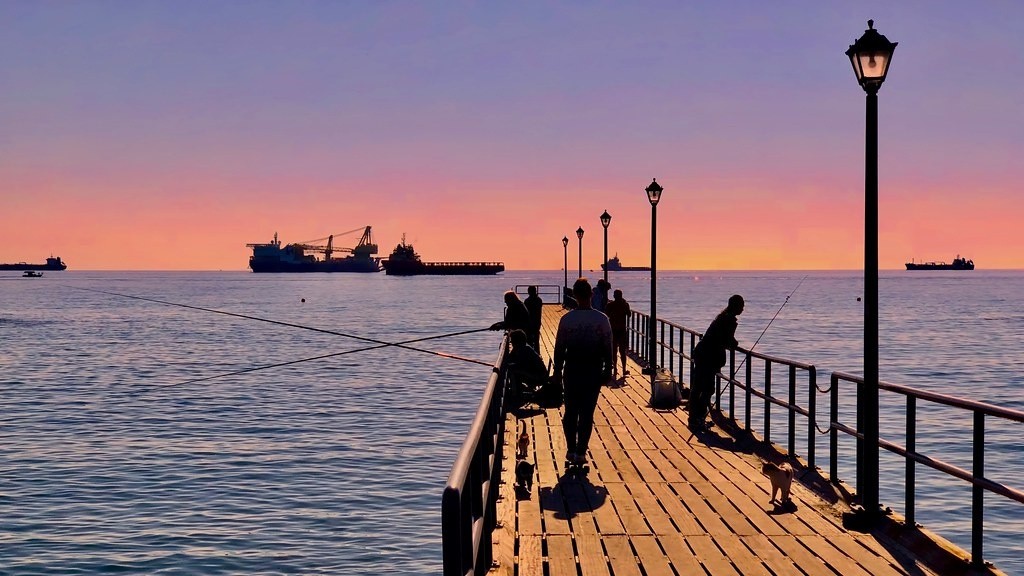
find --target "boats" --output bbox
[380,232,505,276]
[599,250,655,272]
[21,270,43,277]
[244,226,383,273]
[905,252,975,271]
[0,253,71,271]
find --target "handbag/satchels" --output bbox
[533,376,563,408]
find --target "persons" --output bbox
[501,329,548,403]
[553,278,614,463]
[523,286,542,355]
[489,291,530,342]
[687,295,745,431]
[591,280,609,312]
[604,289,632,376]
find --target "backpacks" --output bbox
[649,367,682,410]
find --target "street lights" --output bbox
[645,177,665,382]
[575,226,585,278]
[599,210,613,281]
[562,237,572,292]
[842,21,899,525]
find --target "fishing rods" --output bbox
[685,270,811,446]
[56,279,507,377]
[147,322,503,397]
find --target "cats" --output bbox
[515,459,536,491]
[761,460,794,503]
[518,420,530,458]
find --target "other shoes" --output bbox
[622,370,630,375]
[565,447,589,464]
[687,420,716,437]
